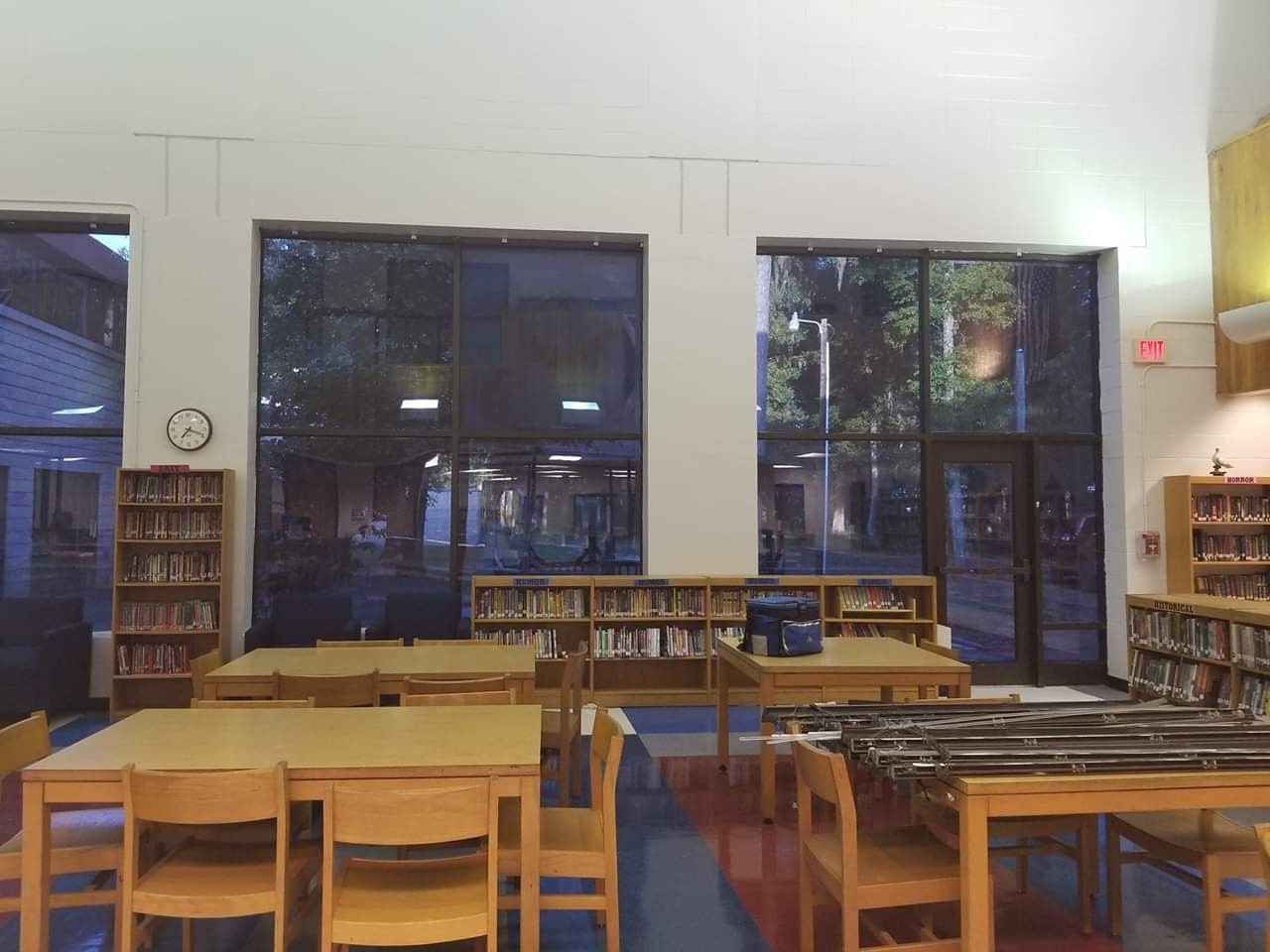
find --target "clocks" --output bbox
[166,408,212,451]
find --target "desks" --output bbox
[924,770,1270,952]
[203,647,536,706]
[717,637,972,823]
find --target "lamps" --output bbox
[1218,301,1270,345]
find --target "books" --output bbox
[1190,494,1270,602]
[116,472,222,674]
[836,586,908,610]
[1126,605,1270,723]
[839,623,885,637]
[751,586,818,599]
[473,587,745,660]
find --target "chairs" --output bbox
[916,638,960,700]
[0,638,624,952]
[0,596,93,715]
[244,591,359,653]
[364,594,471,648]
[786,694,1270,952]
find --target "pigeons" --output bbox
[1211,447,1236,469]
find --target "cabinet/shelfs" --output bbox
[1124,593,1270,719]
[1210,122,1269,396]
[850,483,1063,559]
[110,468,235,724]
[1164,476,1270,594]
[19,704,543,952]
[469,574,938,707]
